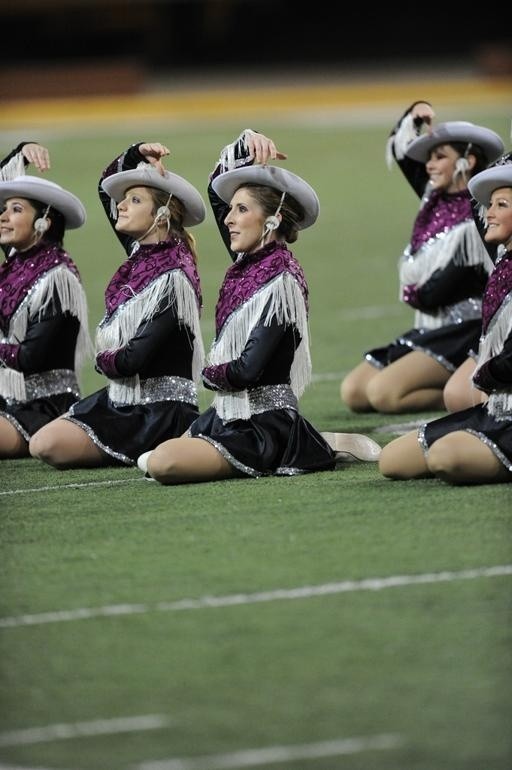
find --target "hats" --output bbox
[101,161,206,228]
[211,162,319,231]
[1,175,86,230]
[467,163,512,209]
[403,121,504,162]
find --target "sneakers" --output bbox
[319,431,382,463]
[137,450,154,475]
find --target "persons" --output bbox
[0,139,93,459]
[28,139,209,477]
[440,348,487,412]
[134,126,381,487]
[339,98,507,416]
[375,150,510,487]
